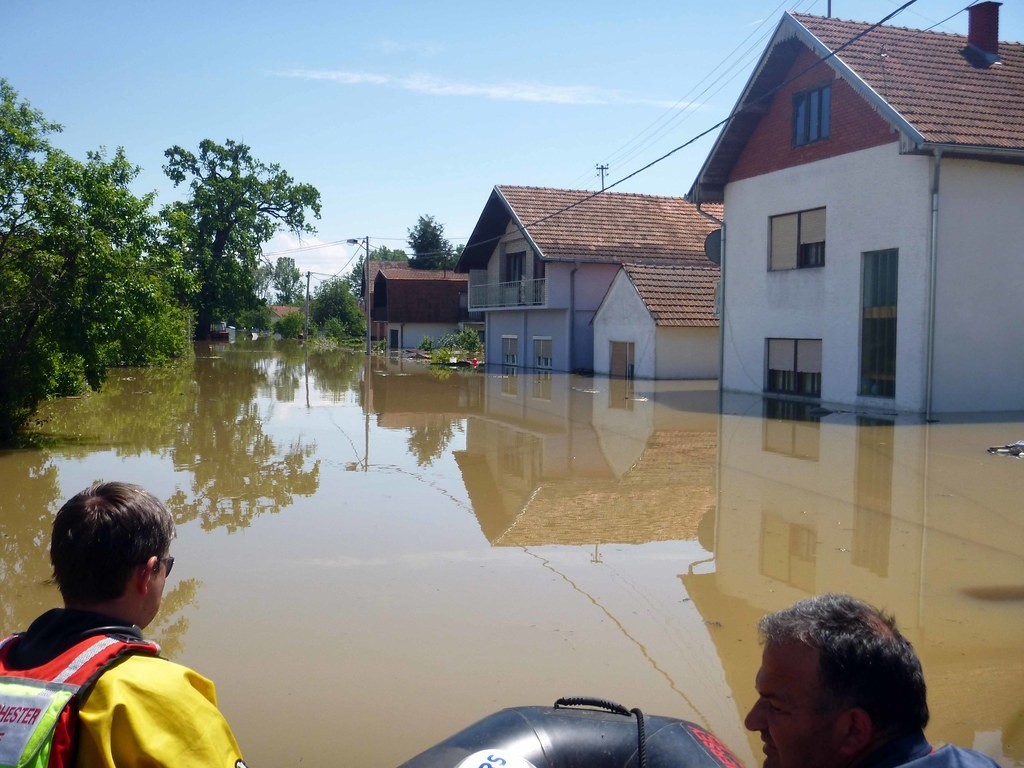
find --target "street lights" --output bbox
[347,236,371,355]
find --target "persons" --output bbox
[0,479,247,768]
[744,591,1002,768]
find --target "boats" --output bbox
[210,321,230,339]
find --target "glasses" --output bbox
[153,555,175,577]
